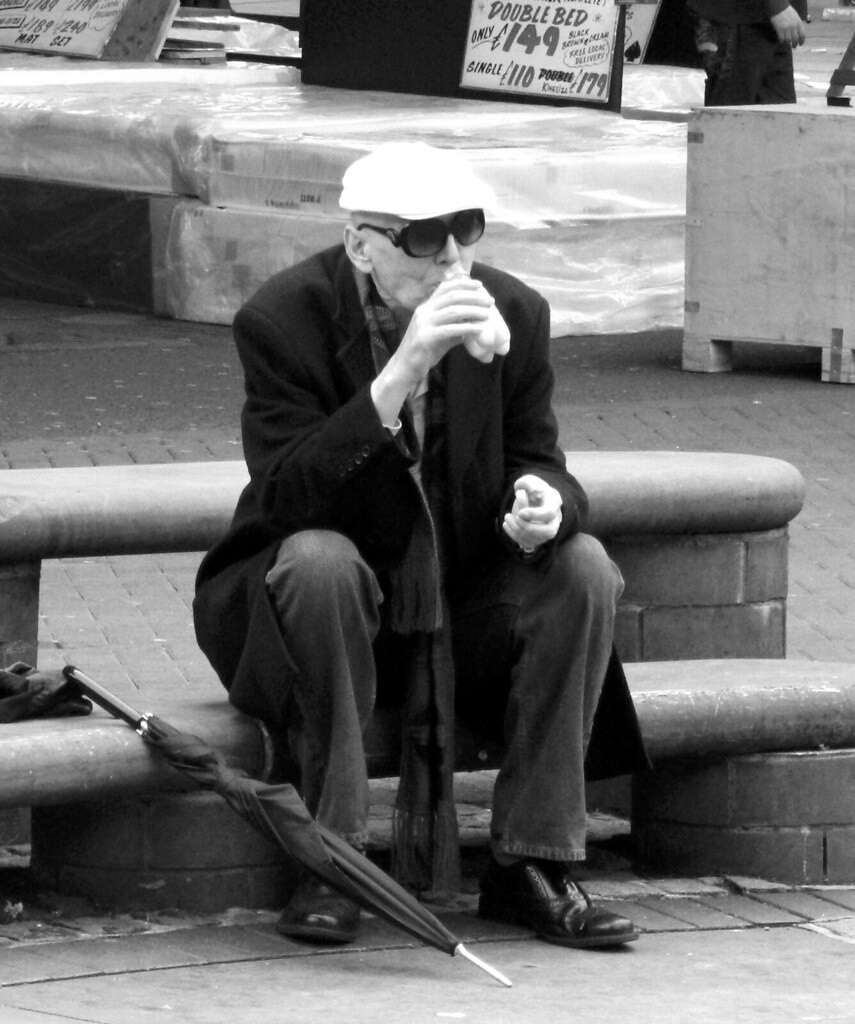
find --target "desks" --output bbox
[684,107,854,384]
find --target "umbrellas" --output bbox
[62,666,517,988]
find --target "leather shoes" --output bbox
[477,857,639,947]
[279,876,362,945]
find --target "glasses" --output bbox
[357,209,485,258]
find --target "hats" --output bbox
[339,142,497,220]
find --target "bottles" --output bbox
[445,264,511,363]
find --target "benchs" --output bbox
[1,451,855,914]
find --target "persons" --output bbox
[193,144,653,949]
[689,0,808,108]
